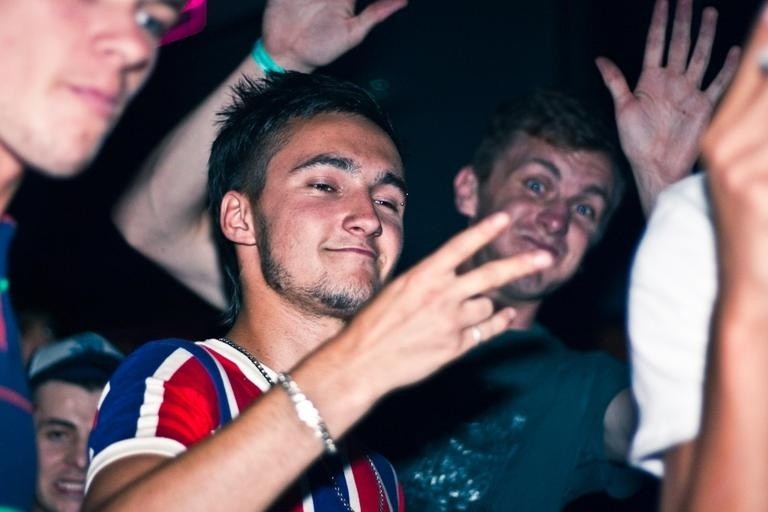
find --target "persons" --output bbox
[2,2,766,511]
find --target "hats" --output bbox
[26,332,125,389]
